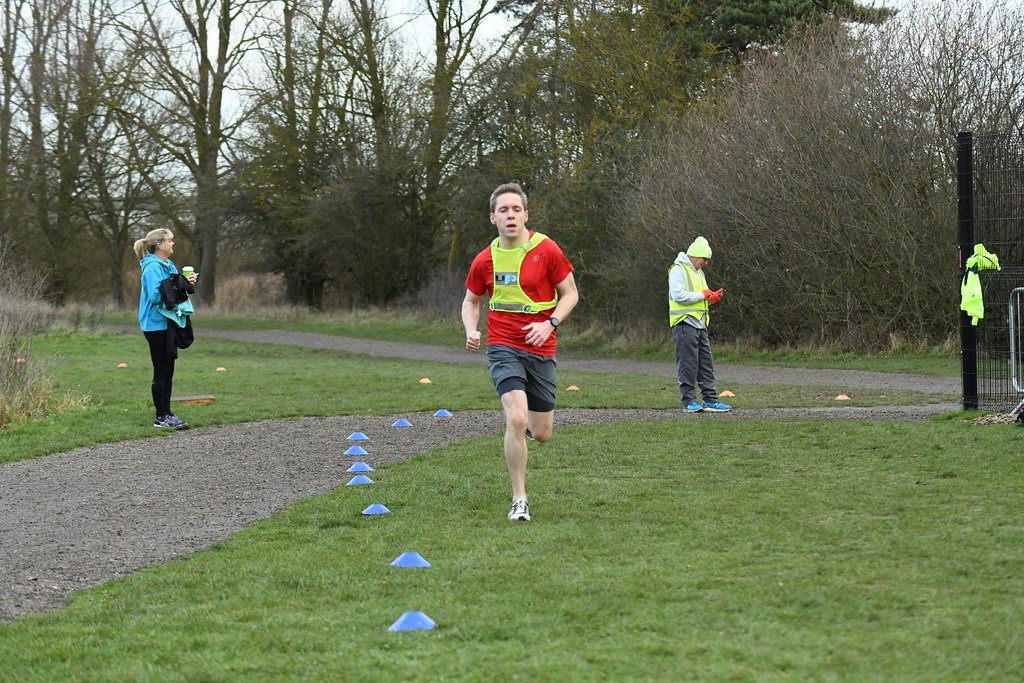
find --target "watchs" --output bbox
[547,317,560,331]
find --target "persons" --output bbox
[134,229,199,429]
[460,184,579,522]
[668,235,733,413]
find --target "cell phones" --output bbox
[717,289,727,297]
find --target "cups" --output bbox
[182,266,195,278]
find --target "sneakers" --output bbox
[702,401,732,412]
[683,401,703,412]
[155,416,188,430]
[524,429,534,440]
[508,497,532,521]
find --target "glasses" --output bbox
[703,257,708,261]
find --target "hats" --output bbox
[687,235,712,258]
